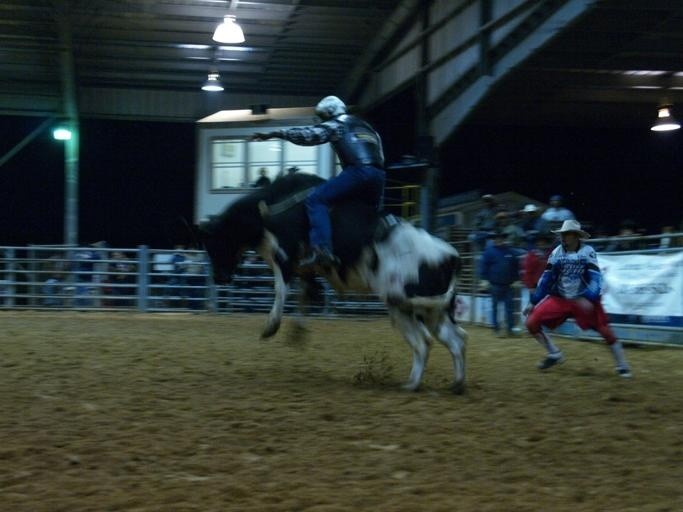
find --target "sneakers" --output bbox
[617,365,631,377]
[536,349,562,369]
[298,248,341,274]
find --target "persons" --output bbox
[473,193,645,340]
[244,95,387,268]
[522,220,632,377]
[256,167,271,185]
[41,242,202,308]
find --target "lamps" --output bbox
[647,90,682,133]
[201,50,224,93]
[212,1,245,45]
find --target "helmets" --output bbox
[314,95,347,118]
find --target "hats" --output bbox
[481,194,591,238]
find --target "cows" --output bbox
[175,173,469,395]
